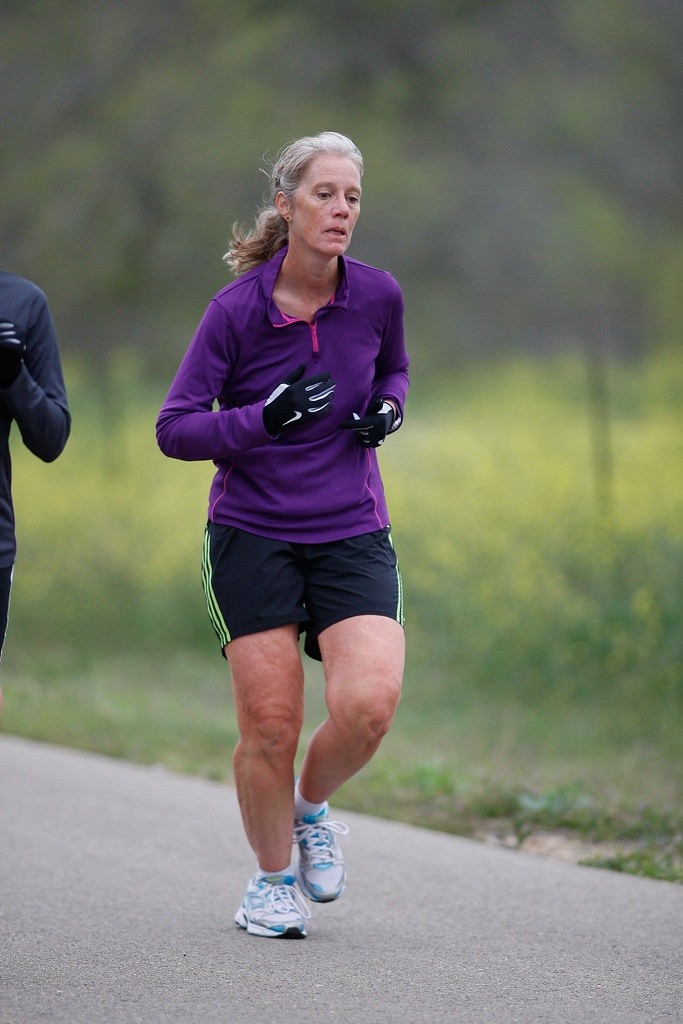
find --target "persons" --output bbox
[1,269,73,656]
[156,130,411,939]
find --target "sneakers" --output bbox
[235,874,312,939]
[293,775,348,903]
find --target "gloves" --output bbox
[0,318,27,389]
[340,398,394,447]
[263,364,337,435]
